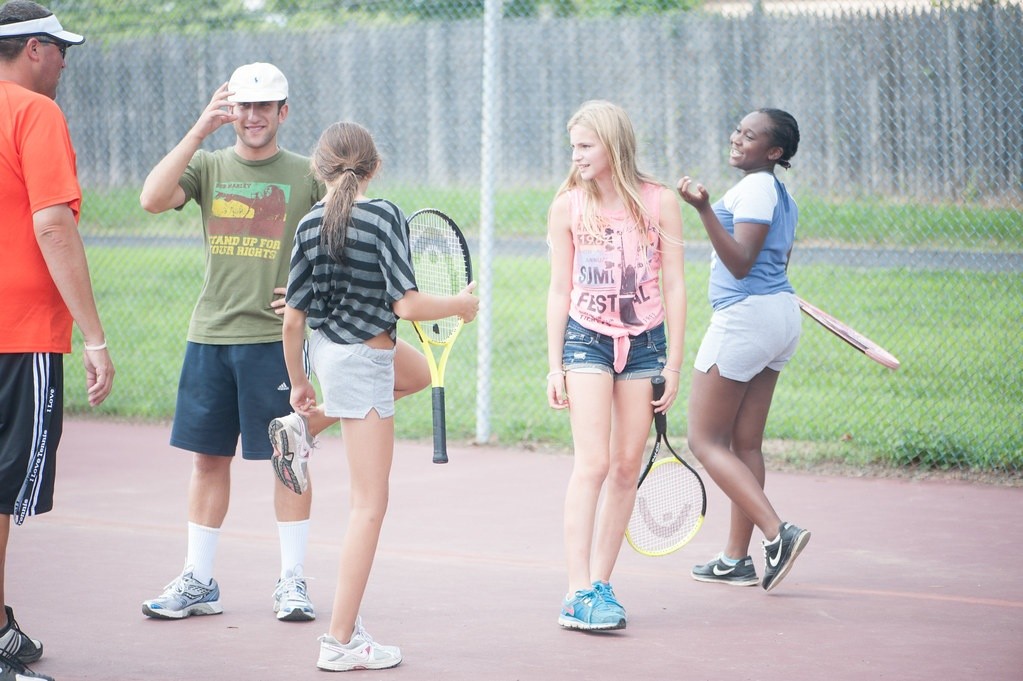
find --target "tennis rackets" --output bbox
[794,294,901,372]
[623,374,710,560]
[405,206,472,466]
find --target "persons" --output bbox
[545,100,687,629]
[268,121,480,671]
[678,109,811,592]
[140,62,326,622]
[0,0,115,681]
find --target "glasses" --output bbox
[22,39,66,60]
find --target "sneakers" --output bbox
[316,615,403,672]
[272,564,316,623]
[761,521,811,591]
[141,557,223,619]
[0,605,43,664]
[691,555,759,587]
[558,587,626,630]
[268,410,323,495]
[593,579,628,617]
[0,648,57,681]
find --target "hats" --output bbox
[227,62,289,102]
[0,13,86,45]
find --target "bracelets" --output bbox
[85,340,107,351]
[547,370,565,379]
